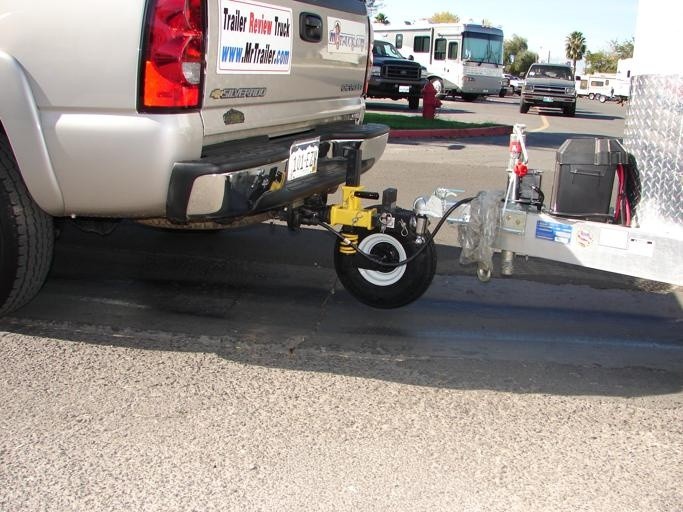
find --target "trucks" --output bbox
[576,59,630,107]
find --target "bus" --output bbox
[361,21,507,102]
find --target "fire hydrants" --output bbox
[418,78,444,120]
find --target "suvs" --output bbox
[366,39,429,110]
[518,62,576,116]
[0,0,391,328]
[502,73,522,96]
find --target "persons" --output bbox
[556,71,564,78]
